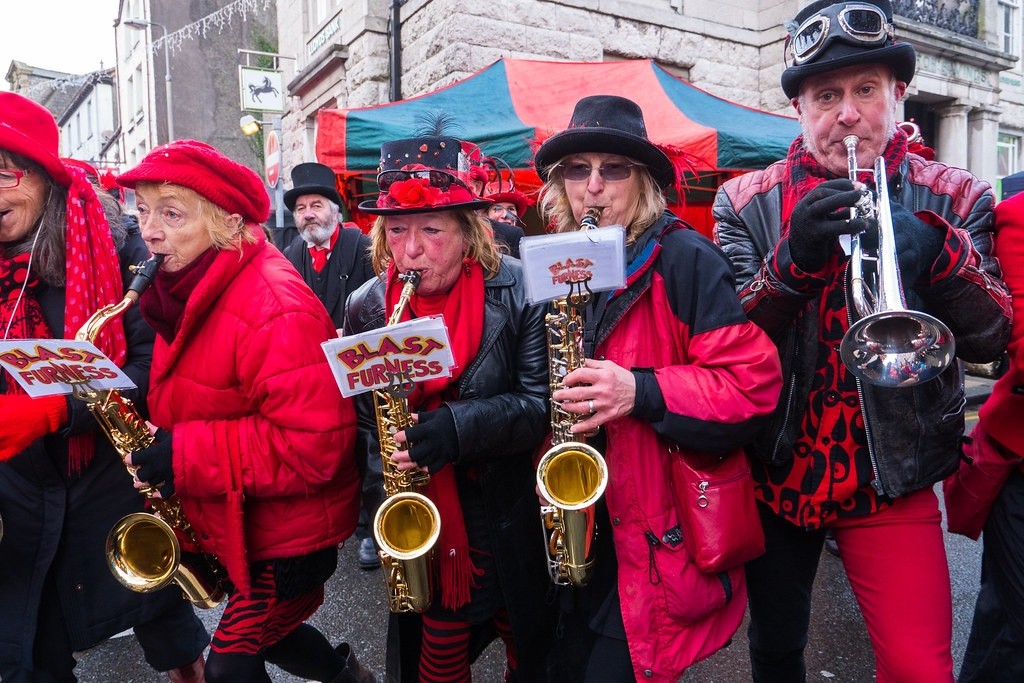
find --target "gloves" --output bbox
[788,178,867,273]
[868,200,943,286]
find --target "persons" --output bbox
[343,105,579,683]
[0,90,210,683]
[279,162,385,567]
[533,97,784,683]
[114,140,378,683]
[712,1,1014,683]
[943,189,1024,683]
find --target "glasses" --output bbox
[559,161,636,181]
[377,169,473,197]
[790,1,894,65]
[0,167,39,187]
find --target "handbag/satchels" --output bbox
[673,448,767,572]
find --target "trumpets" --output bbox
[837,133,956,393]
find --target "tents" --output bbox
[315,57,804,245]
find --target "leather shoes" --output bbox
[358,537,382,567]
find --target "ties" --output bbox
[309,224,340,272]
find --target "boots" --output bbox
[323,641,377,683]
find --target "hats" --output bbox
[781,0,916,101]
[483,181,527,219]
[358,111,491,215]
[284,162,343,213]
[115,139,271,222]
[535,95,674,191]
[0,91,71,185]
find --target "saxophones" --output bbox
[365,269,443,617]
[536,203,614,589]
[67,250,228,613]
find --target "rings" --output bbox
[589,400,594,413]
